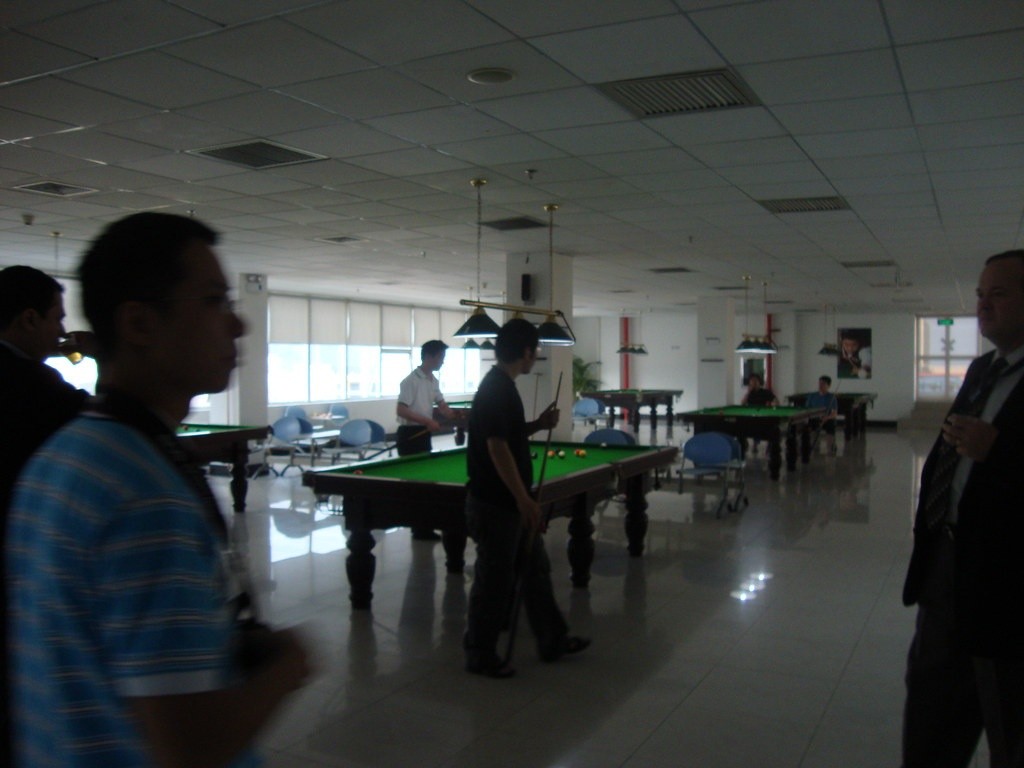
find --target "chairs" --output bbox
[270,398,751,518]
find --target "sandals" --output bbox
[466,656,516,676]
[542,636,590,663]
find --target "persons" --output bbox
[396,340,465,540]
[461,318,594,678]
[841,330,872,376]
[903,249,1024,768]
[0,210,313,768]
[741,373,780,407]
[798,376,838,457]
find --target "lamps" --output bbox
[818,304,842,356]
[734,276,779,354]
[617,308,648,355]
[452,178,577,350]
[247,275,264,294]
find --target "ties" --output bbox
[924,357,1008,532]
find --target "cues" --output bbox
[811,393,836,450]
[502,372,562,665]
[358,410,471,462]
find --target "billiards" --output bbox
[183,425,188,430]
[553,446,561,453]
[574,449,580,456]
[532,452,538,458]
[600,443,607,447]
[579,450,586,457]
[547,450,555,457]
[719,411,724,416]
[559,451,565,457]
[699,412,704,414]
[354,470,363,475]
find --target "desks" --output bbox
[302,440,679,626]
[175,422,269,512]
[432,401,474,446]
[312,414,344,427]
[676,405,828,482]
[786,392,877,441]
[575,388,683,434]
